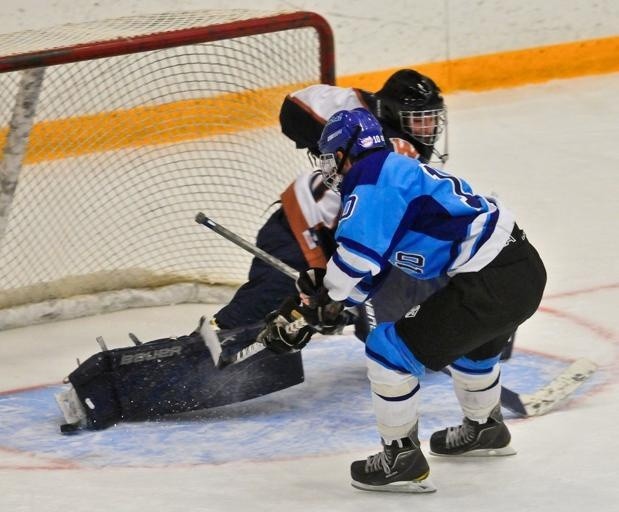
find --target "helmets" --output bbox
[385,69,449,165]
[318,107,386,196]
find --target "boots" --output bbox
[350,420,430,486]
[430,400,511,455]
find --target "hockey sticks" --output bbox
[194,211,596,418]
[199,316,310,369]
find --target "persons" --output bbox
[58,68,450,437]
[255,105,549,485]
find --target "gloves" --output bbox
[262,267,343,357]
